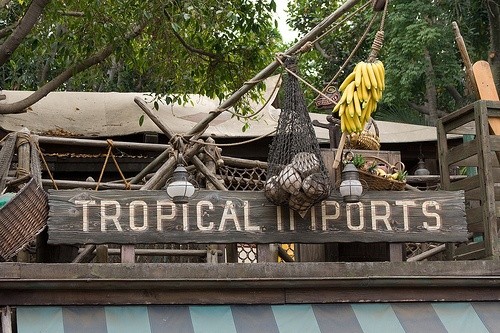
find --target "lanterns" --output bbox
[414,159,430,176]
[160,163,200,204]
[330,161,369,203]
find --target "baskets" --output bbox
[0,175,50,262]
[356,156,407,191]
[345,117,380,150]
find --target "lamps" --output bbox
[339,158,363,203]
[163,152,200,204]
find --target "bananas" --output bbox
[372,168,399,181]
[332,60,385,135]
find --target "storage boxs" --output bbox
[340,150,407,191]
